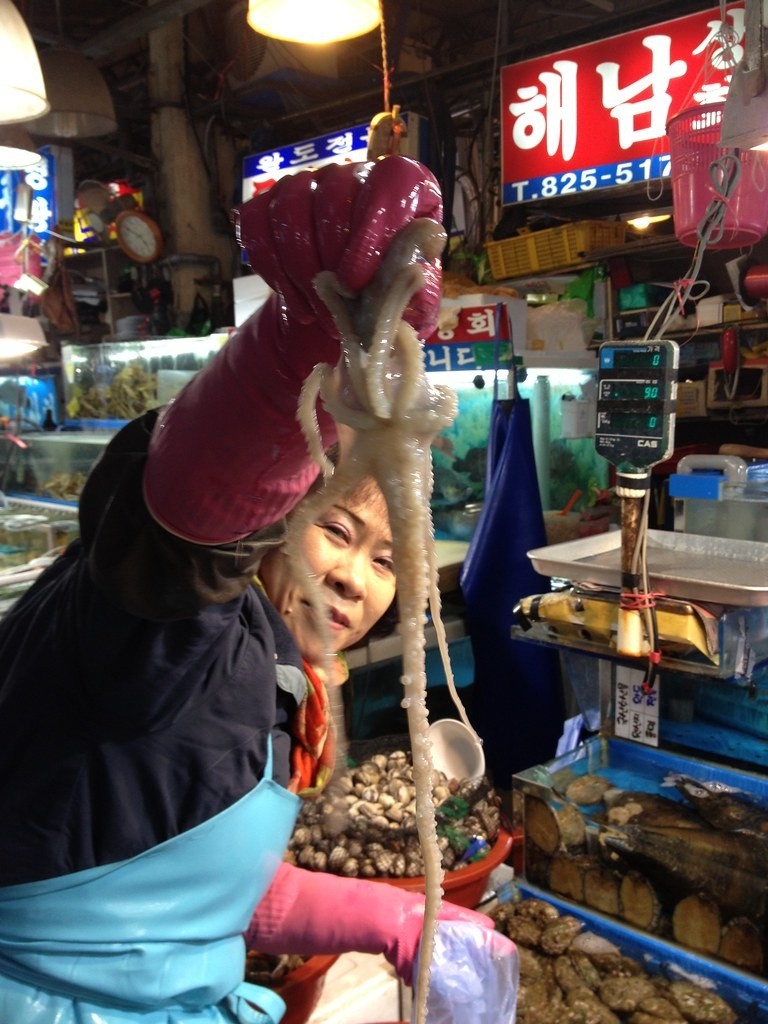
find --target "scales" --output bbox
[526,341,768,685]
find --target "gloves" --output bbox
[148,154,445,545]
[245,863,517,988]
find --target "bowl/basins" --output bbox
[363,829,513,909]
[244,951,340,1024]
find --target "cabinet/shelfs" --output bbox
[603,232,768,437]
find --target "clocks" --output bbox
[116,210,163,263]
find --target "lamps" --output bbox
[0,314,49,359]
[0,0,118,167]
[246,0,383,45]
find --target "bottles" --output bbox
[562,393,577,435]
[150,289,167,336]
[213,285,223,328]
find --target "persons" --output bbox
[0,153,516,1024]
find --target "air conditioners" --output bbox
[223,3,337,94]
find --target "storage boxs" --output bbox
[301,366,768,1024]
[706,365,768,410]
[482,221,627,283]
[0,333,238,620]
[619,283,670,308]
[620,306,663,338]
[667,292,733,332]
[676,379,708,417]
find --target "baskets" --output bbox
[482,220,629,279]
